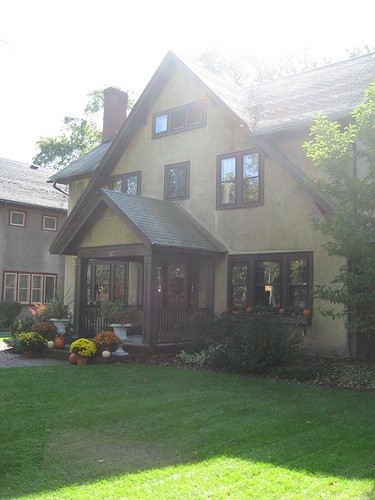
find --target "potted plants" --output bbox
[99,296,143,342]
[246,303,256,314]
[42,298,73,336]
[47,333,55,349]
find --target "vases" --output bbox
[23,348,33,359]
[54,335,68,349]
[69,354,78,364]
[303,306,312,316]
[101,350,111,358]
[279,308,287,315]
[76,355,90,366]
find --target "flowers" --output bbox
[70,337,98,357]
[31,320,58,338]
[17,331,48,351]
[92,330,122,353]
